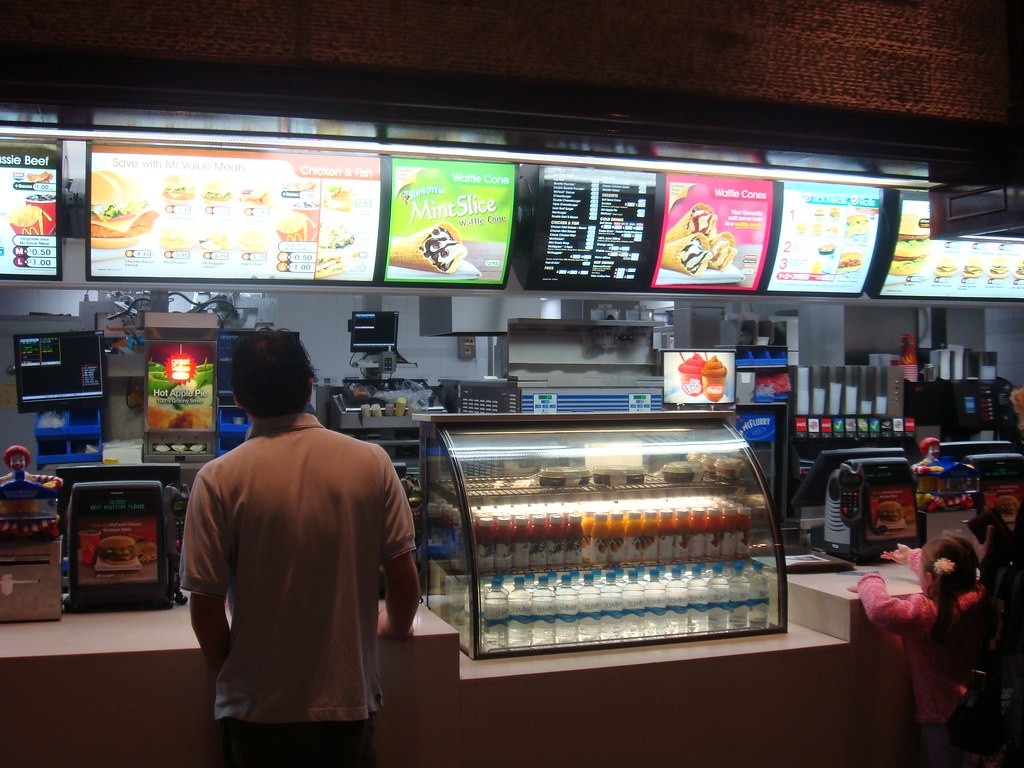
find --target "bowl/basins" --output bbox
[756,337,770,345]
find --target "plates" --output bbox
[877,518,907,530]
[94,557,143,572]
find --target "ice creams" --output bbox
[389,222,467,274]
[708,231,738,271]
[665,202,718,243]
[661,233,710,276]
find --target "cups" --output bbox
[685,451,744,483]
[371,404,382,416]
[590,307,604,320]
[898,332,919,382]
[604,308,619,320]
[79,530,101,565]
[924,362,935,382]
[385,404,394,416]
[293,207,320,241]
[625,309,640,321]
[639,309,655,321]
[679,373,726,403]
[361,404,371,416]
[25,199,56,234]
[395,397,407,416]
[870,497,879,525]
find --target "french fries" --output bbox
[277,213,305,233]
[10,204,40,226]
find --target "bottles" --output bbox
[449,569,605,650]
[670,335,674,349]
[600,560,771,643]
[436,502,584,572]
[580,494,752,567]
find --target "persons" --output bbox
[910,438,944,511]
[961,388,1024,768]
[847,535,991,768]
[179,328,420,768]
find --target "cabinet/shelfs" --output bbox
[410,411,789,661]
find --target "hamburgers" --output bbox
[838,253,862,273]
[797,207,840,235]
[889,214,930,276]
[198,225,232,252]
[162,222,196,249]
[161,175,194,204]
[239,176,273,208]
[879,501,902,525]
[936,258,958,277]
[91,171,159,248]
[202,180,232,204]
[97,535,137,564]
[239,228,273,252]
[989,257,1010,278]
[847,216,869,239]
[1014,258,1024,279]
[962,259,983,277]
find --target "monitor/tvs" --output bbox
[938,441,1011,465]
[792,448,904,507]
[350,311,398,353]
[56,463,181,537]
[13,330,106,414]
